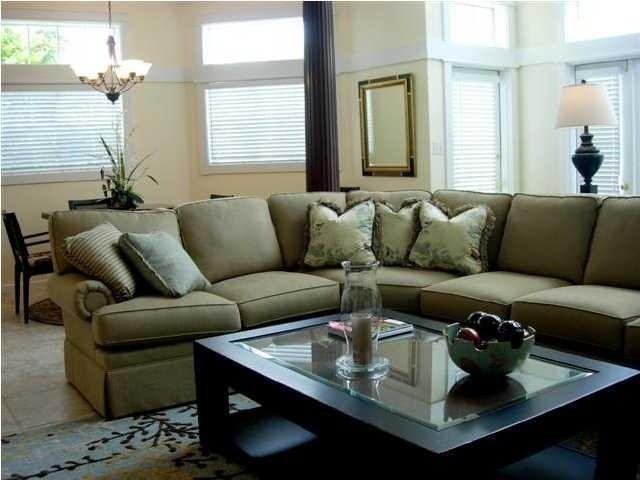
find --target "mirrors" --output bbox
[357,73,418,178]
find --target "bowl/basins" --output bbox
[444,373,526,420]
[442,321,536,375]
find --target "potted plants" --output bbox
[99,114,159,210]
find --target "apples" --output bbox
[456,312,529,349]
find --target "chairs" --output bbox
[1,209,54,323]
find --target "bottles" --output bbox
[344,372,385,404]
[337,260,390,374]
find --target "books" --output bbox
[328,316,414,342]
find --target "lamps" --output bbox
[68,1,152,105]
[555,79,619,194]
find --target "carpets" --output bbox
[30,297,64,326]
[1,390,262,480]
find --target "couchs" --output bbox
[45,189,639,421]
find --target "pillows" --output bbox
[295,195,374,271]
[419,195,494,274]
[121,232,214,298]
[57,224,136,295]
[374,198,417,267]
